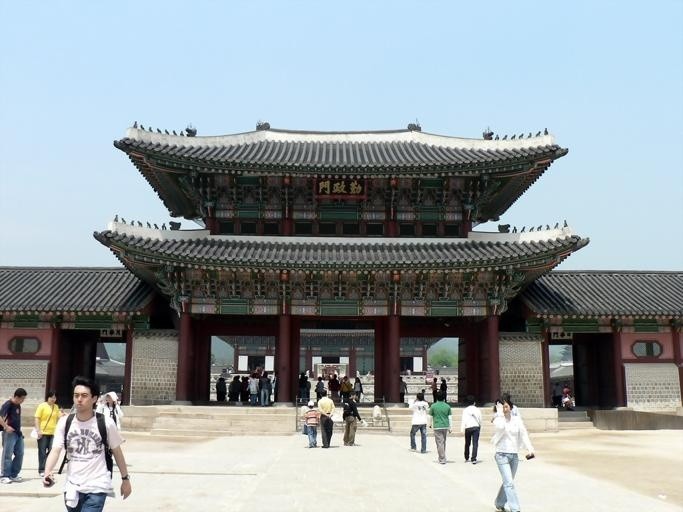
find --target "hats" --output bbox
[307,401,315,407]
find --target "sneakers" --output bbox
[472,459,478,464]
[39,471,45,477]
[407,447,449,465]
[495,503,507,512]
[10,476,23,482]
[0,476,12,484]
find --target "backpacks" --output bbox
[64,412,115,481]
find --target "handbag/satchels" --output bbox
[0,423,5,432]
[302,423,309,435]
[30,428,42,440]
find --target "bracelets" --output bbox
[3,425,7,430]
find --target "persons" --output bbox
[41,376,131,511]
[489,395,535,512]
[427,389,452,464]
[299,372,368,448]
[215,372,271,406]
[552,389,563,406]
[429,376,439,400]
[420,388,430,404]
[439,381,449,402]
[562,384,575,397]
[32,390,66,476]
[0,387,27,483]
[95,391,123,479]
[459,397,481,463]
[400,376,408,401]
[562,394,573,407]
[490,393,521,423]
[408,392,430,453]
[491,399,503,415]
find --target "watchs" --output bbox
[121,474,130,480]
